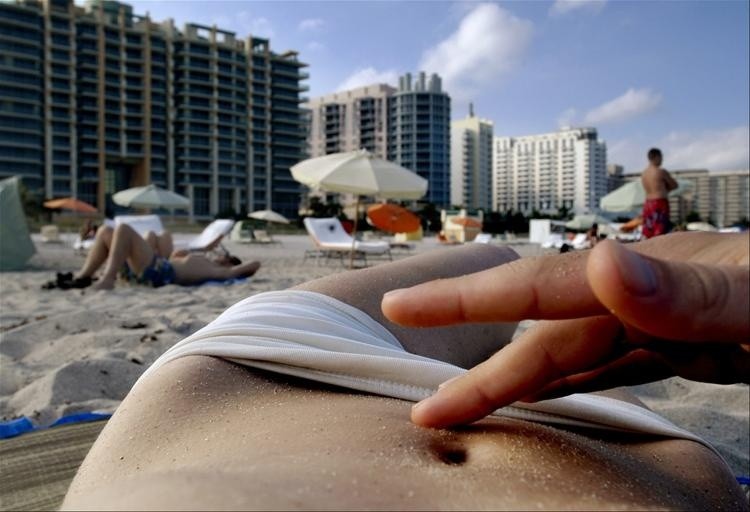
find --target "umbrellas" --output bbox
[291,147,428,270]
[450,215,484,243]
[112,183,190,215]
[43,195,98,213]
[248,209,289,226]
[366,201,422,252]
[600,176,687,214]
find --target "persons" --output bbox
[57,229,750,512]
[586,223,597,245]
[72,222,262,293]
[639,146,679,240]
[144,231,174,257]
[555,227,578,247]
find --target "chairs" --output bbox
[303,215,393,268]
[253,230,284,248]
[474,231,493,244]
[188,218,235,250]
[541,232,592,251]
[114,213,165,240]
